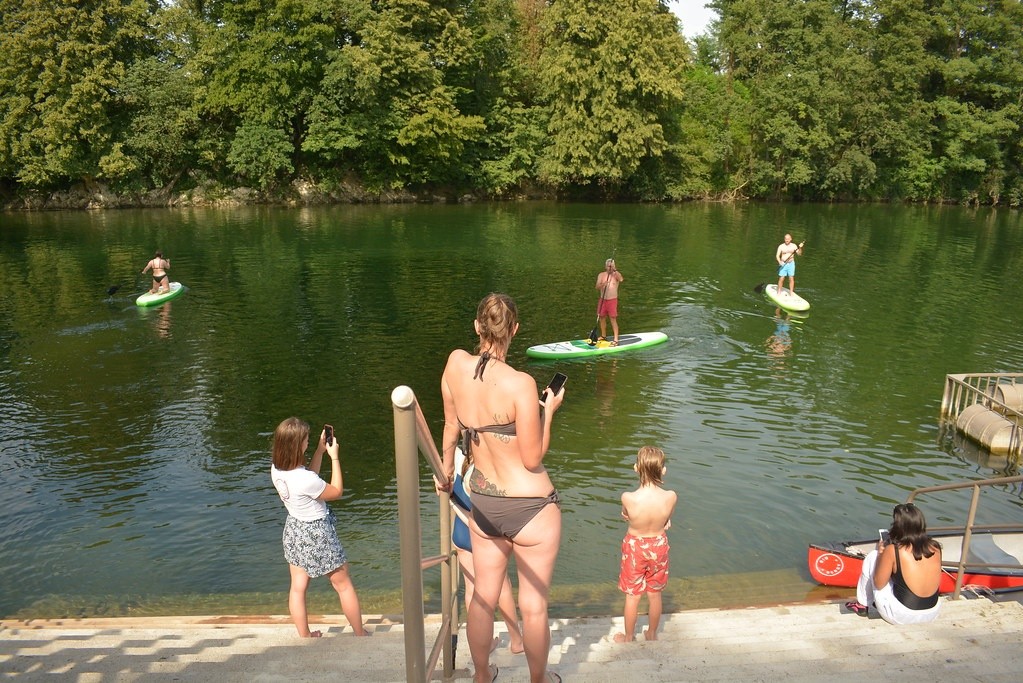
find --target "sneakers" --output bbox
[872,602,876,609]
[845,602,869,617]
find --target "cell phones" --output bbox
[324,424,334,446]
[879,529,889,541]
[539,372,568,407]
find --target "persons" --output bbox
[142,251,171,295]
[433,294,565,683]
[845,503,942,625]
[613,446,677,643]
[270,417,369,637]
[595,259,624,347]
[776,234,803,296]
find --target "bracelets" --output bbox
[614,270,618,272]
[331,459,339,460]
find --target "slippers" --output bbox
[473,666,498,683]
[546,672,563,683]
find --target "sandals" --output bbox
[609,341,619,347]
[597,336,606,340]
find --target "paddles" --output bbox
[587,247,617,347]
[105,274,142,295]
[754,238,808,294]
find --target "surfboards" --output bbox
[765,282,811,313]
[135,281,184,307]
[525,330,669,360]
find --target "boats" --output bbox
[808,522,1023,599]
